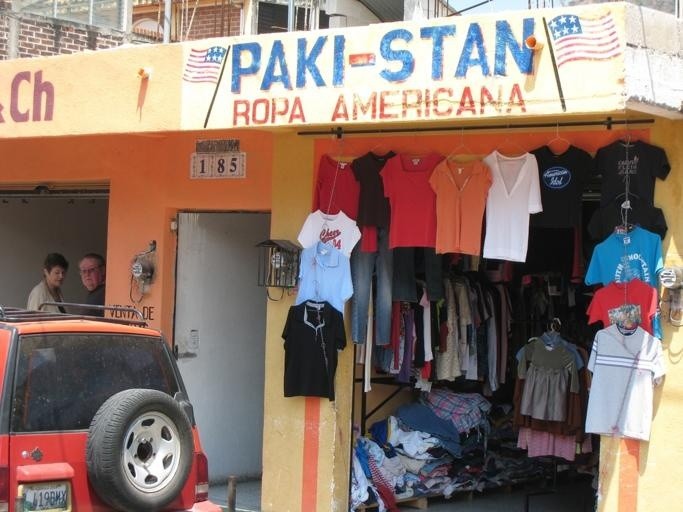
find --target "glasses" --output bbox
[79,264,104,274]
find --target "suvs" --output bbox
[0,294,224,511]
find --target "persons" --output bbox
[77,252,104,316]
[24,252,71,313]
